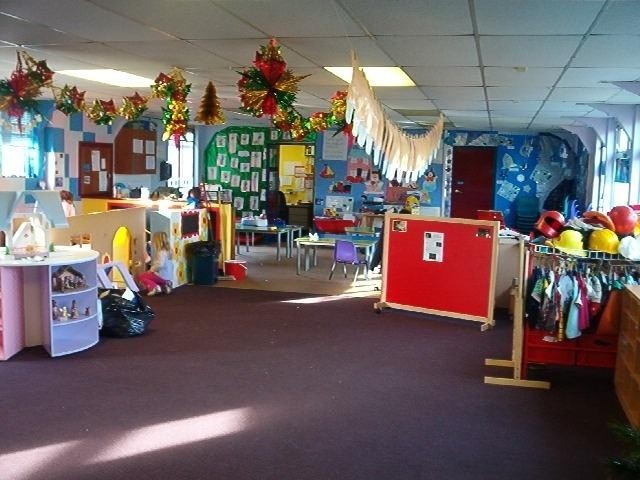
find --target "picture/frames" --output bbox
[235,222,303,262]
[294,236,377,276]
[306,233,381,240]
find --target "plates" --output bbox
[612,284,640,434]
[0,247,99,362]
[521,325,617,380]
[289,205,311,229]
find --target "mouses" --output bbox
[537,255,636,279]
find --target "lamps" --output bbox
[474,209,505,231]
[515,192,541,234]
[329,240,368,282]
[265,190,289,224]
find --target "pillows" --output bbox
[535,204,640,262]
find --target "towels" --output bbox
[186,240,222,286]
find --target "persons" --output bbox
[60,191,75,217]
[137,232,174,296]
